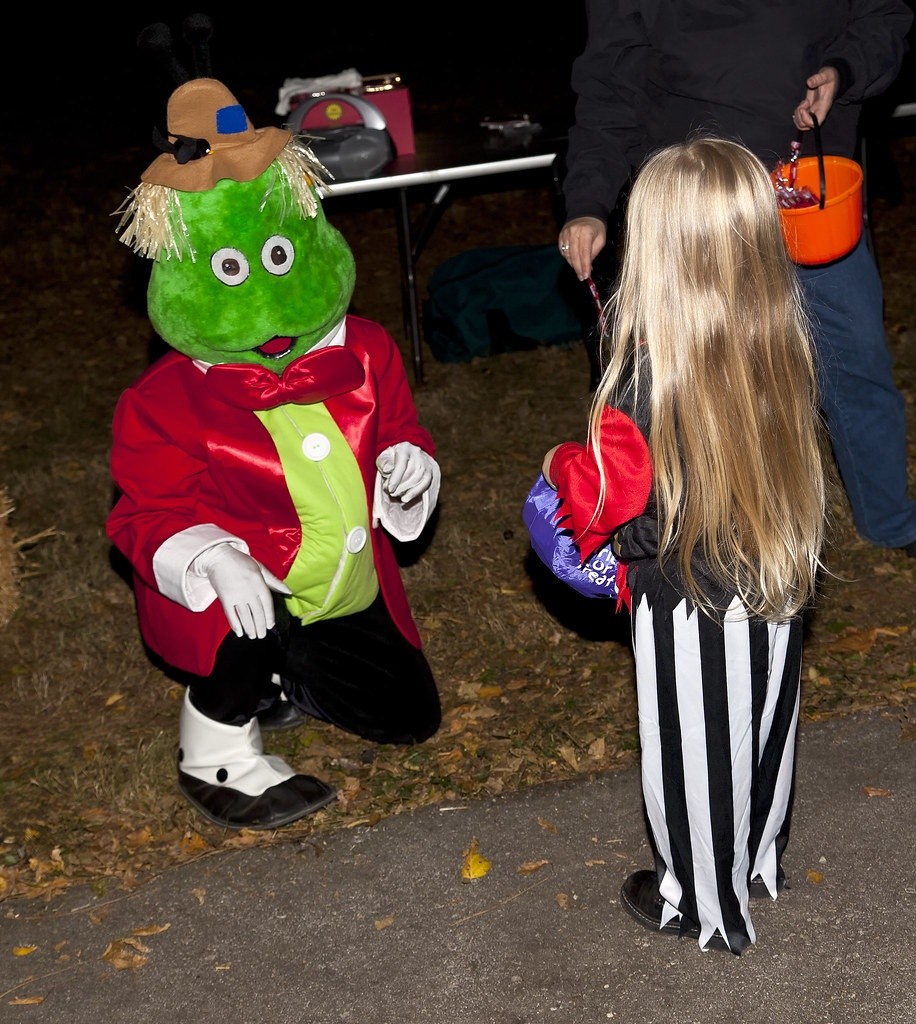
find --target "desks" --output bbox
[313,153,558,383]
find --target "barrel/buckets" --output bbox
[767,154,864,266]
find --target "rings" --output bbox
[562,244,569,250]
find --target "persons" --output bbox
[559,0,916,562]
[541,138,826,958]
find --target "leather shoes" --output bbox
[748,863,786,899]
[619,869,731,951]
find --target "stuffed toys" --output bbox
[104,78,442,831]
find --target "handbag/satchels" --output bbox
[521,470,619,599]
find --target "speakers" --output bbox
[286,94,397,182]
